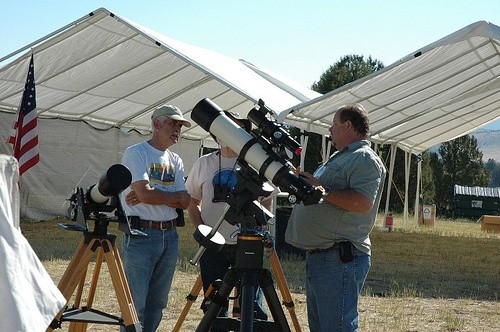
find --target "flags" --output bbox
[6,54,40,175]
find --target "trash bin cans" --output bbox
[418,204,423,224]
[423,204,436,227]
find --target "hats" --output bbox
[210,109,252,143]
[152,105,191,128]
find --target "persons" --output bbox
[119,105,192,332]
[0,152,67,332]
[284,104,386,332]
[184,111,274,332]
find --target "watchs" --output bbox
[324,186,332,195]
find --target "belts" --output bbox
[139,221,177,231]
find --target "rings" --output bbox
[133,198,137,202]
